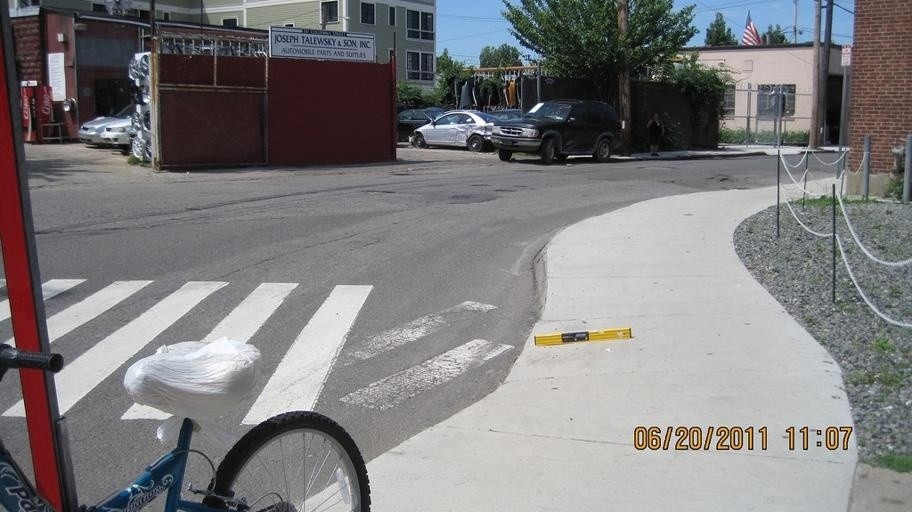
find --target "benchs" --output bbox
[741,12,762,47]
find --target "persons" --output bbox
[646,112,680,157]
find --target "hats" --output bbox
[77,100,137,156]
[397,98,627,164]
